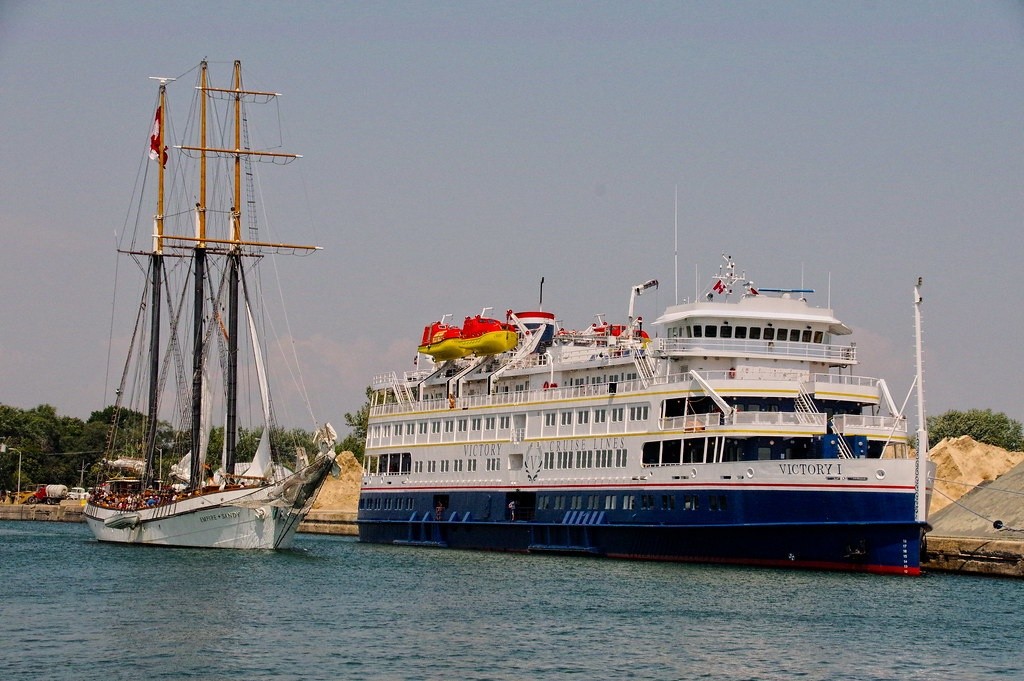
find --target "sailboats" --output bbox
[82,57,343,551]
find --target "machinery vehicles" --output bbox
[5,483,68,506]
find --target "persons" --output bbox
[89,464,246,511]
[33,485,46,501]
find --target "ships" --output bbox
[356,182,933,576]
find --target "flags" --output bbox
[148,105,168,169]
[713,280,726,295]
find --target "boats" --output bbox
[416,314,473,362]
[459,306,518,357]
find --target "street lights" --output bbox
[9,447,21,505]
